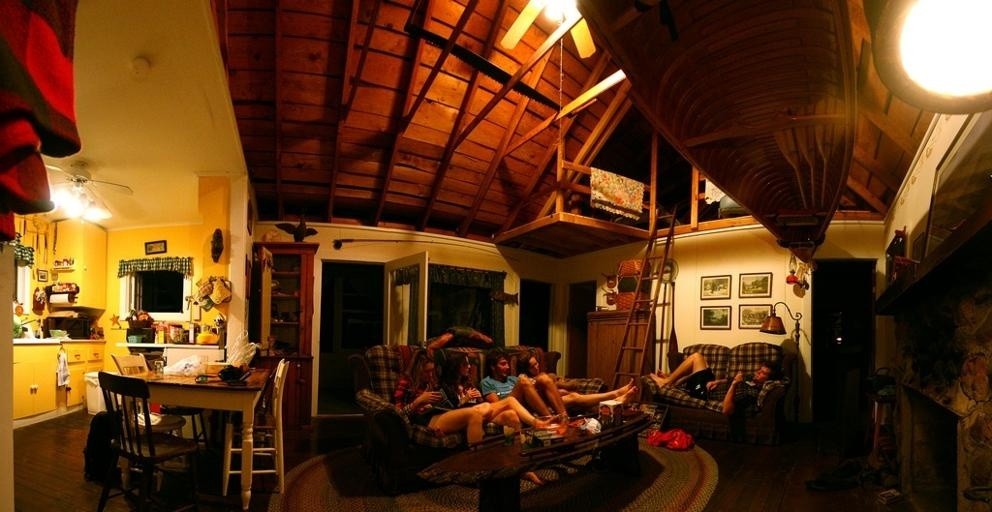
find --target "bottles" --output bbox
[34,325,44,339]
[153,320,220,345]
[54,258,68,268]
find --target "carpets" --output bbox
[269,431,719,512]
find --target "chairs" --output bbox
[223,357,291,497]
[138,350,209,449]
[111,351,186,437]
[96,370,197,512]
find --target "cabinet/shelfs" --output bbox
[57,339,107,421]
[10,333,61,433]
[247,240,321,458]
[585,310,657,402]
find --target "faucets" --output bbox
[19,316,37,326]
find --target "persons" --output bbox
[649,351,782,417]
[393,347,492,444]
[439,351,561,488]
[479,348,569,421]
[514,351,640,411]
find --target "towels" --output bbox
[57,353,71,386]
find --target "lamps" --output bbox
[760,297,803,445]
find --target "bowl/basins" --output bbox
[206,361,232,374]
[127,319,153,328]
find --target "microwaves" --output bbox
[46,316,92,338]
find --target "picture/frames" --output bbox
[739,303,775,330]
[144,239,166,255]
[36,269,48,282]
[700,275,732,300]
[738,272,773,297]
[698,305,732,332]
[919,113,992,263]
[913,232,925,261]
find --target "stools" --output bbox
[863,391,898,458]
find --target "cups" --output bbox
[155,360,164,379]
[504,426,515,447]
[520,428,533,448]
[199,354,209,374]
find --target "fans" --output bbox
[42,156,135,226]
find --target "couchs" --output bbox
[348,343,608,494]
[638,334,795,449]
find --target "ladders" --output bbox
[609,204,678,393]
